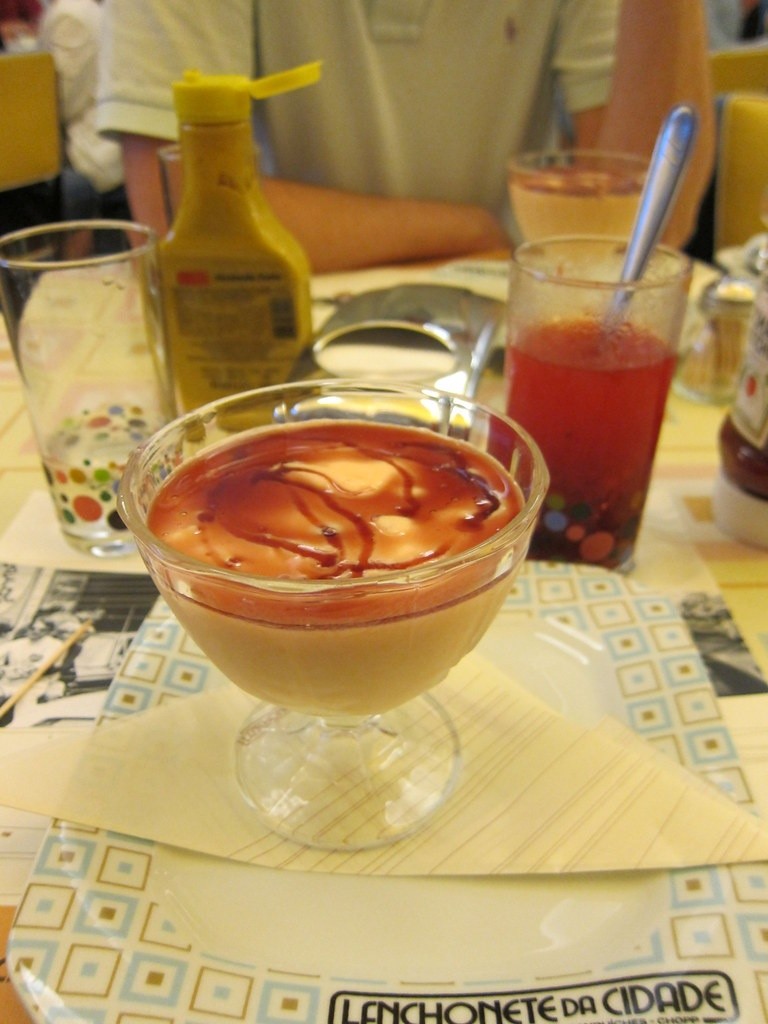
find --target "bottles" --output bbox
[138,58,323,426]
[712,265,768,553]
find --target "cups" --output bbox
[0,216,173,559]
[510,234,695,574]
[151,141,196,228]
[671,260,758,403]
[503,150,649,253]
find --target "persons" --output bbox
[94,0,716,276]
[0,1,121,261]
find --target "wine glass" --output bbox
[117,376,550,851]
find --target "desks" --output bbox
[0,245,768,1024]
